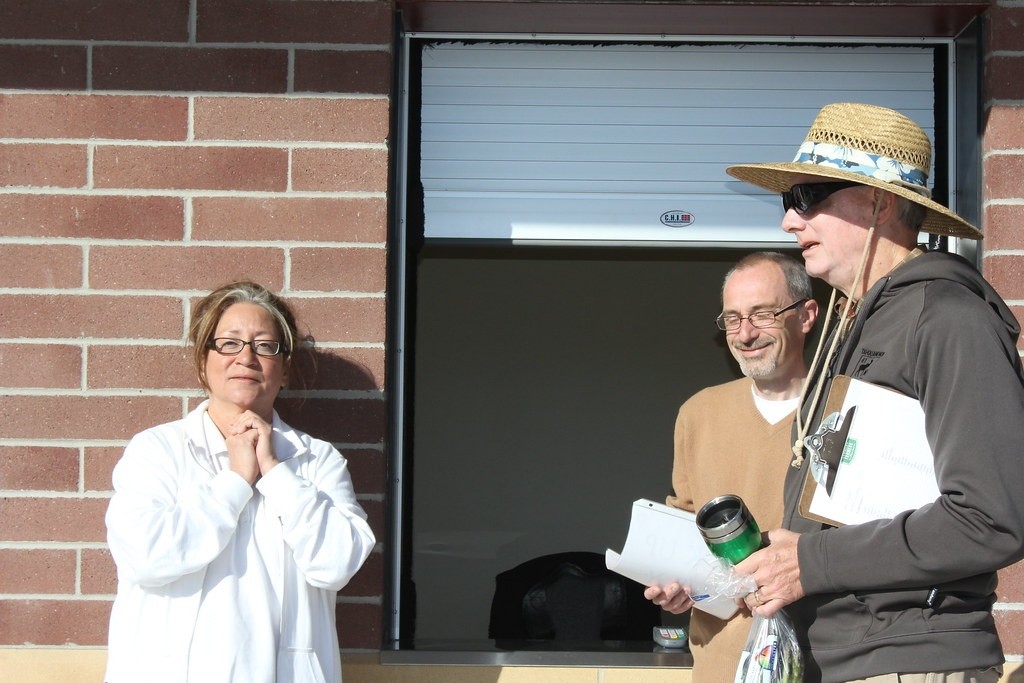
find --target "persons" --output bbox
[105,278,376,683]
[725,104,1024,683]
[644,251,818,683]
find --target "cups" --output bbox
[696,494,767,567]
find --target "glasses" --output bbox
[780,181,865,217]
[205,337,287,356]
[714,297,809,331]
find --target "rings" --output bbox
[755,591,765,605]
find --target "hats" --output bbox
[726,103,986,241]
